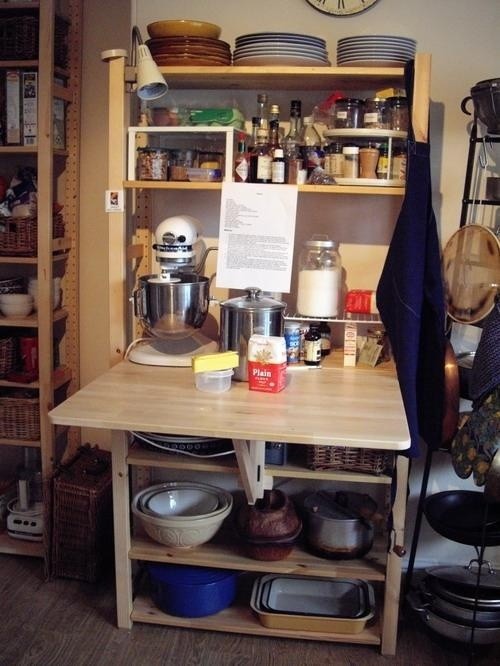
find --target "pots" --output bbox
[424,489,500,544]
[221,287,285,381]
[461,77,499,133]
[303,492,373,557]
[409,562,500,640]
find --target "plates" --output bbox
[234,34,331,65]
[338,36,416,65]
[129,431,237,458]
[149,21,230,66]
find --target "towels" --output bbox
[462,303,499,410]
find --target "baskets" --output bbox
[0,337,18,380]
[306,444,396,476]
[0,8,68,62]
[0,398,64,440]
[1,215,66,255]
[48,446,113,583]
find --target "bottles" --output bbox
[234,130,249,182]
[268,103,282,158]
[322,141,330,175]
[256,90,269,139]
[329,141,343,178]
[295,232,341,317]
[390,143,407,178]
[282,98,305,183]
[341,142,360,178]
[333,97,365,129]
[360,95,385,129]
[377,142,390,180]
[303,323,321,365]
[283,322,301,364]
[136,144,173,181]
[384,93,410,131]
[248,113,262,182]
[270,147,286,183]
[250,139,272,183]
[168,147,199,180]
[299,114,323,178]
[320,319,331,356]
[195,146,224,180]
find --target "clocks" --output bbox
[305,0,381,20]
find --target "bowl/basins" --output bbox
[2,293,30,319]
[131,481,234,549]
[1,277,26,293]
[26,276,60,312]
[250,574,376,633]
[194,369,232,391]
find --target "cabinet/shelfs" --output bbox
[0,0,84,585]
[109,51,434,658]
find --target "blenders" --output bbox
[127,213,217,369]
